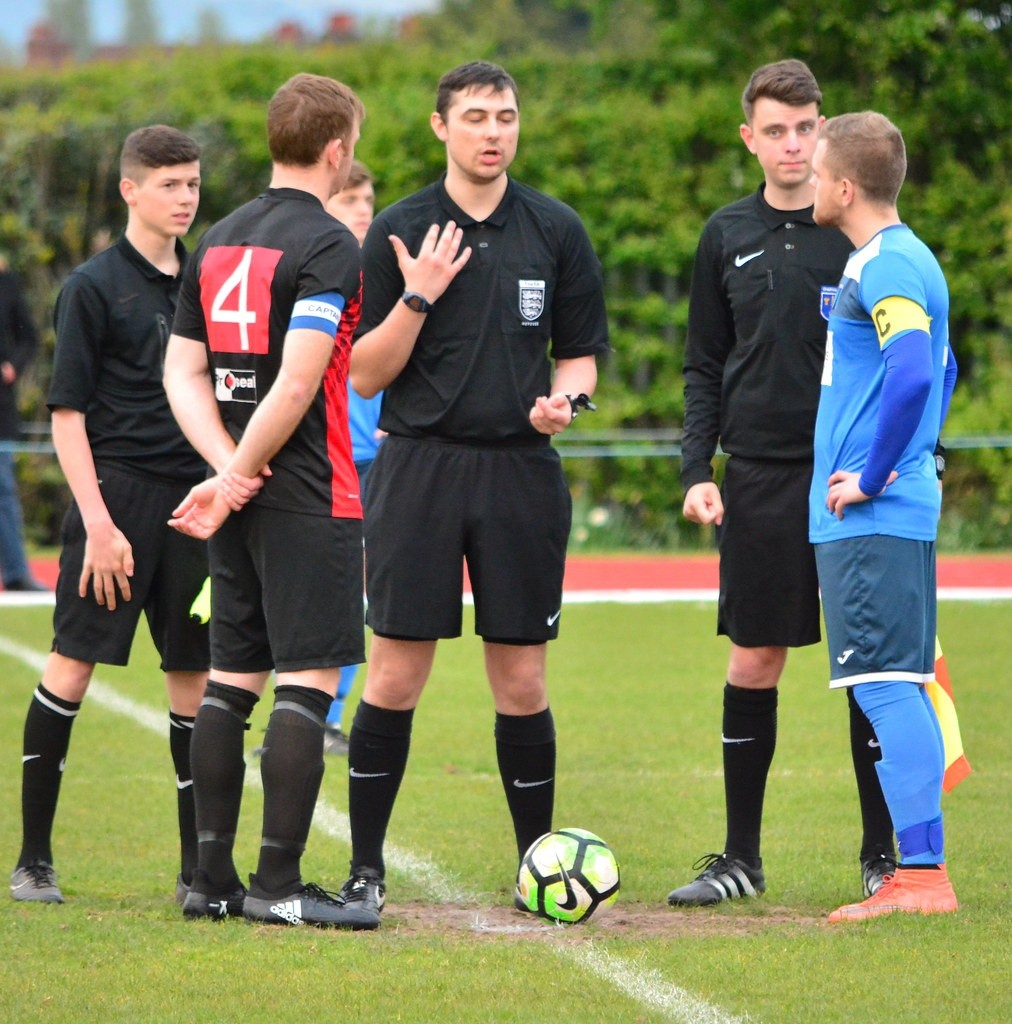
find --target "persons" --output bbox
[161,72,381,932]
[0,265,47,591]
[339,59,612,915]
[810,111,959,922]
[10,122,208,904]
[321,167,389,757]
[665,58,946,905]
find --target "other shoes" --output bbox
[4,576,49,593]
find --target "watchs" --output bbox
[401,290,434,313]
[934,456,945,471]
[566,393,597,426]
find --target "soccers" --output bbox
[515,827,622,928]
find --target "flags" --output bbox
[924,635,976,793]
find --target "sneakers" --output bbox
[828,867,959,924]
[181,869,251,920]
[241,872,381,928]
[323,723,350,755]
[9,859,65,903]
[334,861,388,930]
[859,849,899,898]
[174,871,193,906]
[512,862,563,923]
[665,851,766,907]
